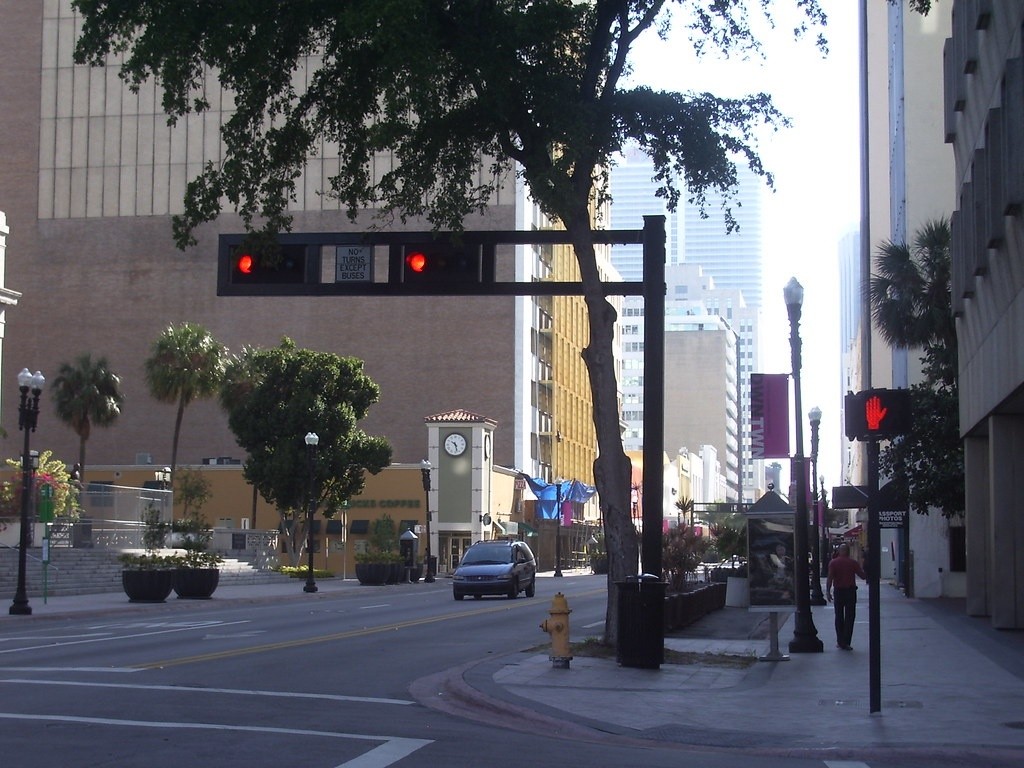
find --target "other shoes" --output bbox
[842,645,853,650]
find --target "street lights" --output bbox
[807,403,828,607]
[420,458,436,584]
[554,475,563,577]
[302,430,320,593]
[783,275,824,653]
[9,368,46,615]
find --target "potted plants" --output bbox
[118,554,179,603]
[355,552,394,587]
[410,559,425,584]
[172,550,224,600]
[388,554,407,586]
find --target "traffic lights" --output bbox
[400,243,478,287]
[859,386,894,436]
[229,247,304,285]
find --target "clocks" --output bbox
[446,433,465,456]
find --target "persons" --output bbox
[69,463,81,490]
[826,541,868,650]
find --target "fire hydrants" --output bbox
[539,592,573,669]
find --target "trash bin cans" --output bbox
[424,555,438,576]
[612,573,670,670]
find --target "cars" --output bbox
[452,538,536,601]
